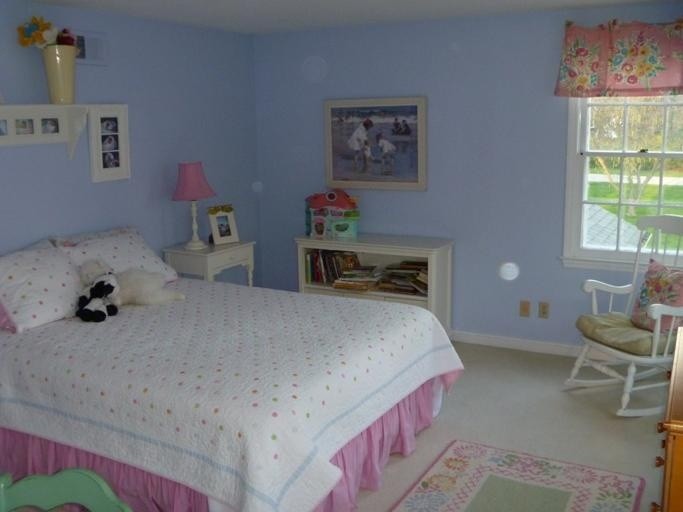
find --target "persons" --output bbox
[217,222,231,237]
[0,117,118,168]
[336,108,411,174]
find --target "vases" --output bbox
[37,42,85,109]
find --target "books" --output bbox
[305,249,428,296]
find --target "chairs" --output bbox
[552,213,683,420]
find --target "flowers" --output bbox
[13,12,98,53]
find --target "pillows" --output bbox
[68,219,181,309]
[0,233,93,336]
[630,258,683,332]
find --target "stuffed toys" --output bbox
[76,273,119,323]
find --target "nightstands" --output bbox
[158,238,262,292]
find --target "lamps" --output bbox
[165,150,223,258]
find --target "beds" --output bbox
[0,220,478,512]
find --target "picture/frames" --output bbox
[318,92,430,196]
[0,100,70,147]
[84,101,134,184]
[205,202,241,246]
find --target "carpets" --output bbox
[377,435,648,511]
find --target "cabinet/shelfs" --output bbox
[289,230,452,343]
[648,327,681,510]
